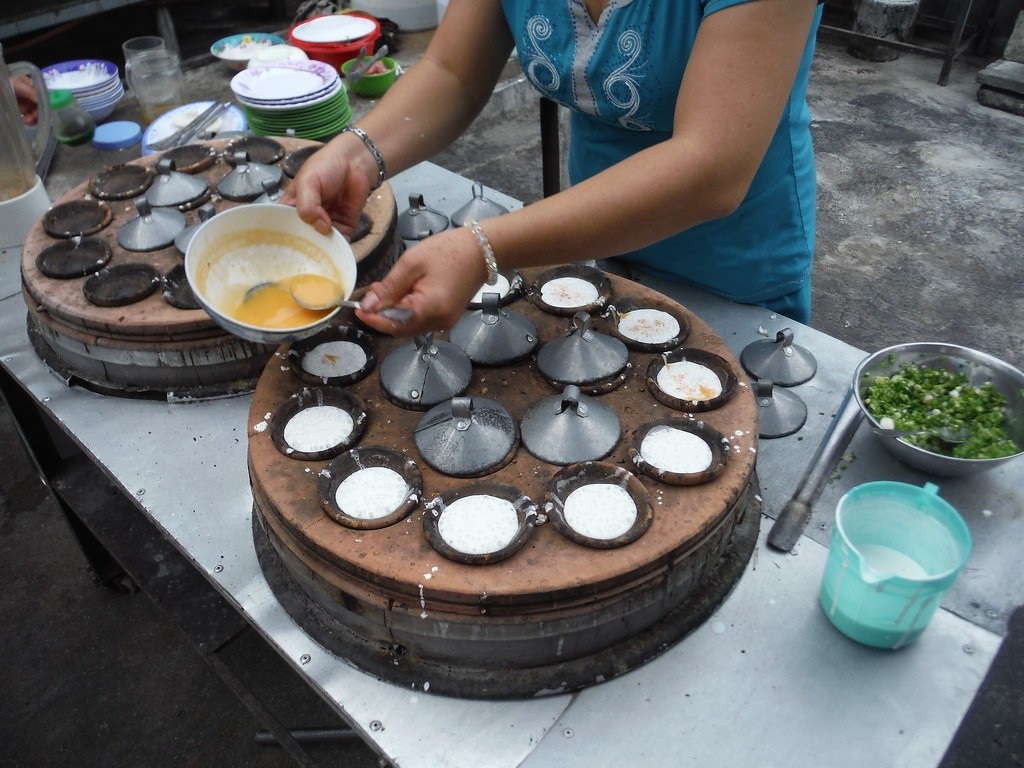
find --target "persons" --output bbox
[278,0,825,340]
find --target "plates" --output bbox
[230,45,352,140]
[292,15,376,42]
[141,101,254,158]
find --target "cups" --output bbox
[122,37,166,99]
[0,174,51,248]
[92,119,142,169]
[134,50,188,124]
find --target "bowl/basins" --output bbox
[39,59,124,122]
[183,203,358,342]
[210,33,285,72]
[341,56,398,98]
[852,342,1024,478]
[289,14,381,78]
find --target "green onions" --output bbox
[861,353,1024,459]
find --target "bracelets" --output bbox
[342,126,386,191]
[464,218,498,286]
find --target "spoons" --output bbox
[871,426,973,443]
[290,274,414,322]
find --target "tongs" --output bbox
[145,101,234,151]
[348,45,388,81]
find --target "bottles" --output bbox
[49,89,95,147]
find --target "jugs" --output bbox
[817,481,972,647]
